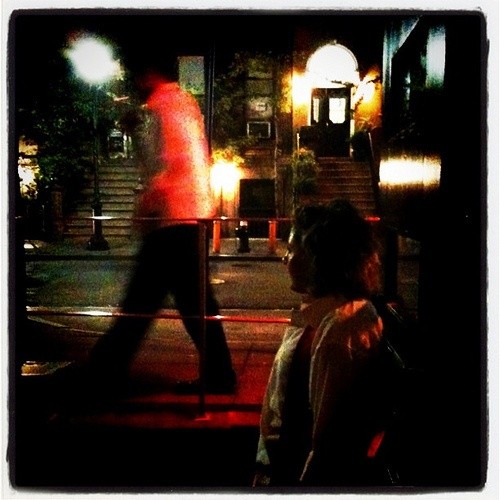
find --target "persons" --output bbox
[83,66,239,396]
[254,197,399,493]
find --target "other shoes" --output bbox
[171,375,236,396]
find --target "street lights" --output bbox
[81,60,126,250]
